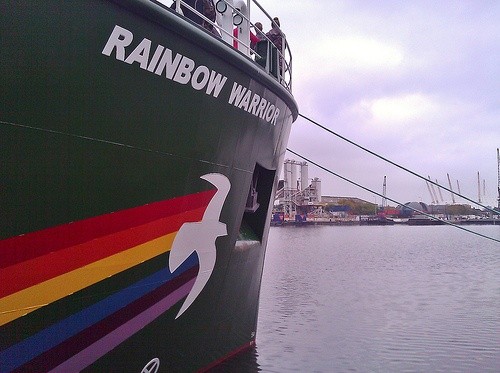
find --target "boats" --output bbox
[0,0,300,373]
[405,218,445,225]
[359,216,395,225]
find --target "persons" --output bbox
[232,25,261,53]
[265,16,287,83]
[170,0,217,34]
[250,22,267,56]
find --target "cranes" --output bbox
[382,176,388,208]
[424,170,487,206]
